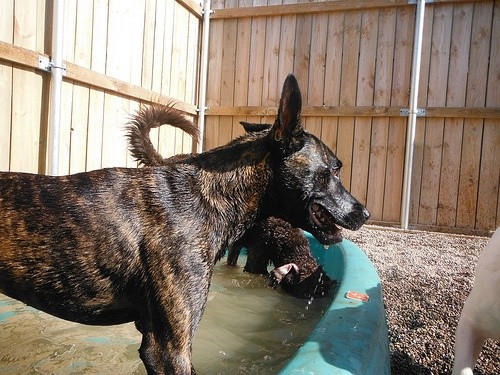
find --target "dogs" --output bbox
[451,225,500,375]
[123,98,337,299]
[0,73,370,375]
